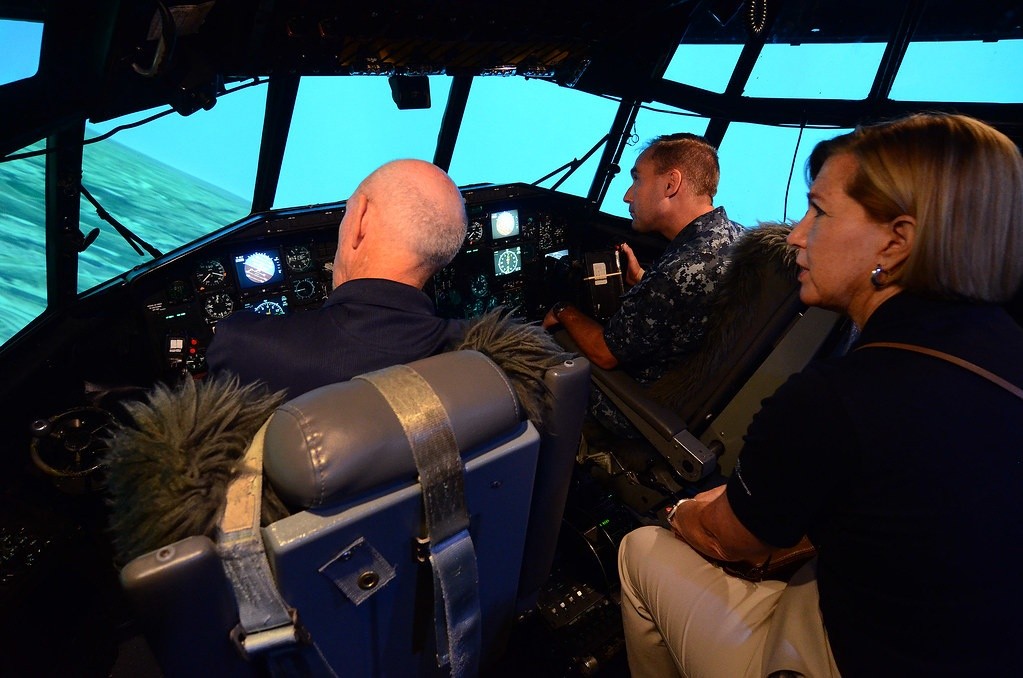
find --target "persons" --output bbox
[613,110,1023,678]
[206,158,483,401]
[542,132,750,445]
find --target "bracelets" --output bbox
[667,499,696,529]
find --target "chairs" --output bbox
[554,218,854,526]
[110,305,591,678]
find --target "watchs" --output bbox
[553,301,566,317]
